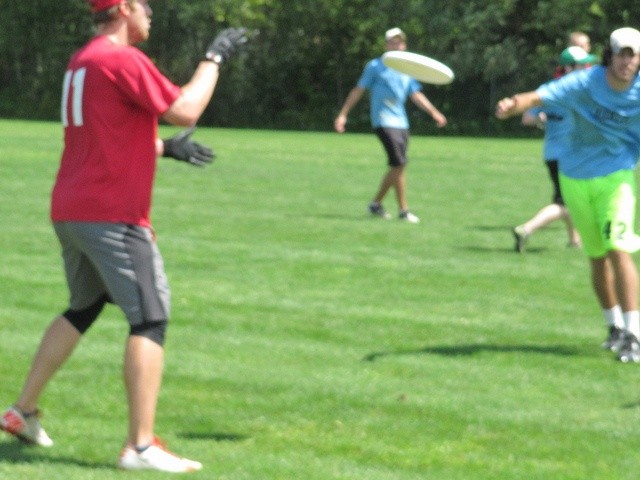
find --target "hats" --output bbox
[560,47,596,66]
[610,27,640,56]
[387,28,407,39]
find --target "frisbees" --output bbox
[380,50,455,86]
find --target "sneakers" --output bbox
[118,436,203,473]
[369,202,388,219]
[396,213,420,223]
[615,333,640,363]
[512,226,527,251]
[604,324,624,350]
[0,405,54,449]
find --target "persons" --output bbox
[334,28,447,224]
[1,1,261,472]
[512,45,600,254]
[554,30,592,80]
[495,27,639,362]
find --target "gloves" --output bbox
[204,27,257,66]
[162,128,216,166]
